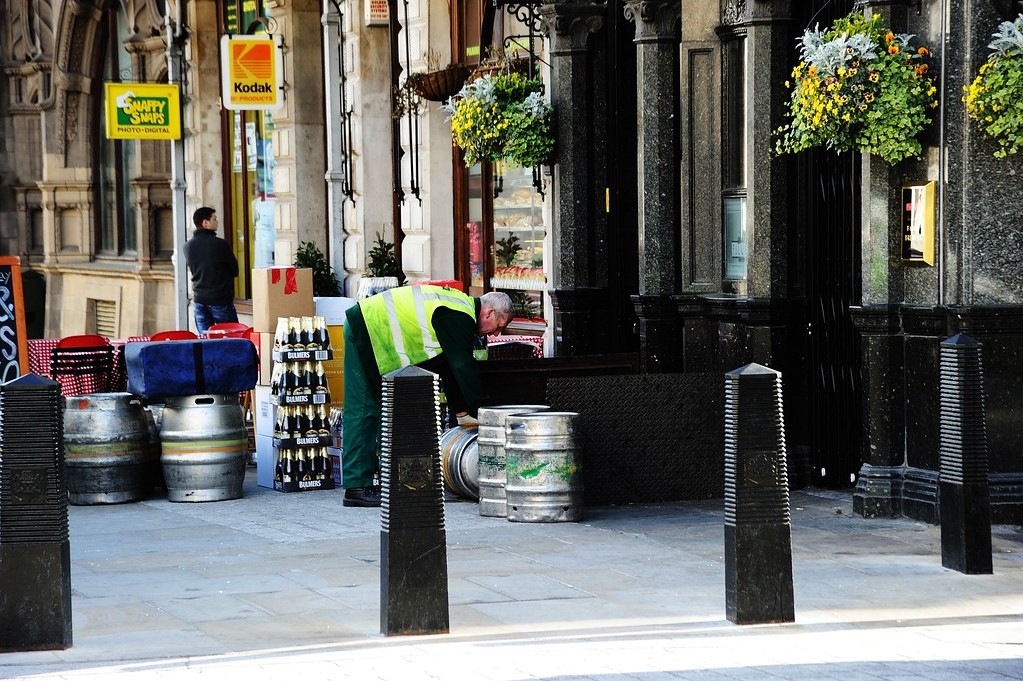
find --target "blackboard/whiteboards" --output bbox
[0,256,29,384]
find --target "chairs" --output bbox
[49,320,249,393]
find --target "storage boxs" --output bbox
[253,267,317,490]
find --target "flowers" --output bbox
[963,16,1023,160]
[450,71,554,167]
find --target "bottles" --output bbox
[271,316,344,483]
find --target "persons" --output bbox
[343,284,514,507]
[182,207,239,334]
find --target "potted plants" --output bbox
[384,46,467,119]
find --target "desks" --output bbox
[27,332,250,397]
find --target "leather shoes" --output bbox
[343,487,382,506]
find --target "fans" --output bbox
[773,12,937,163]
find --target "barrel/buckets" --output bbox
[65,392,248,505]
[505,412,582,522]
[477,404,549,517]
[442,426,479,501]
